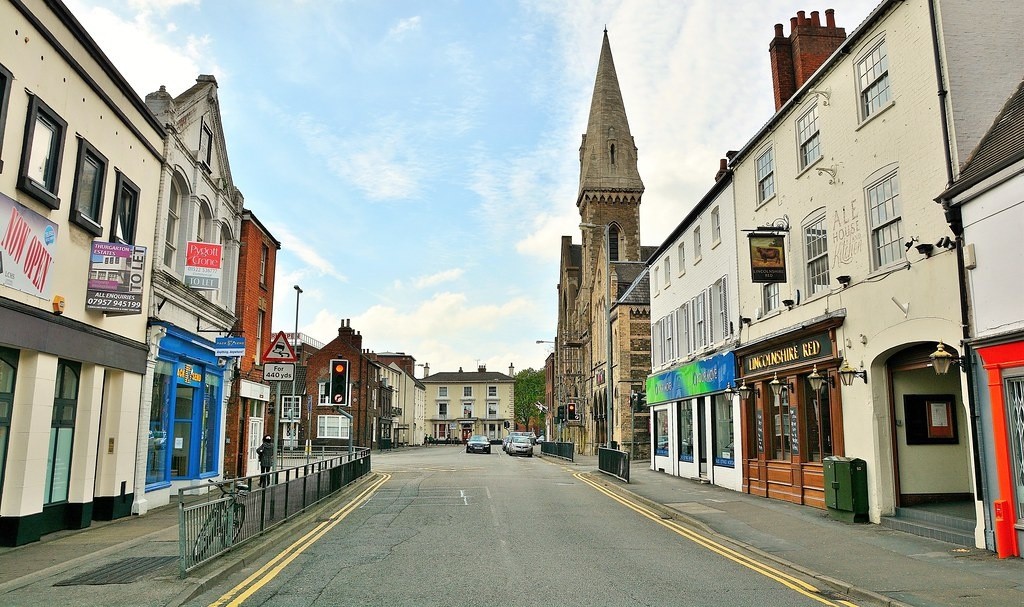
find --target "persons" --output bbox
[423,434,433,447]
[256,435,274,487]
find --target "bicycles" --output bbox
[193,478,250,562]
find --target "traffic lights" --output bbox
[268,402,274,414]
[567,403,576,421]
[637,393,646,413]
[504,421,510,429]
[330,359,349,406]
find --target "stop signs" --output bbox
[629,397,630,407]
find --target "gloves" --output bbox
[260,450,263,452]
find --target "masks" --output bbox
[265,439,271,443]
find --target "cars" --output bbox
[510,432,537,446]
[501,435,516,454]
[536,435,544,444]
[509,436,533,458]
[466,435,491,454]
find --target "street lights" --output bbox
[290,285,303,452]
[403,364,424,447]
[536,341,561,442]
[579,222,613,449]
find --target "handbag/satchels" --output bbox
[258,444,264,461]
